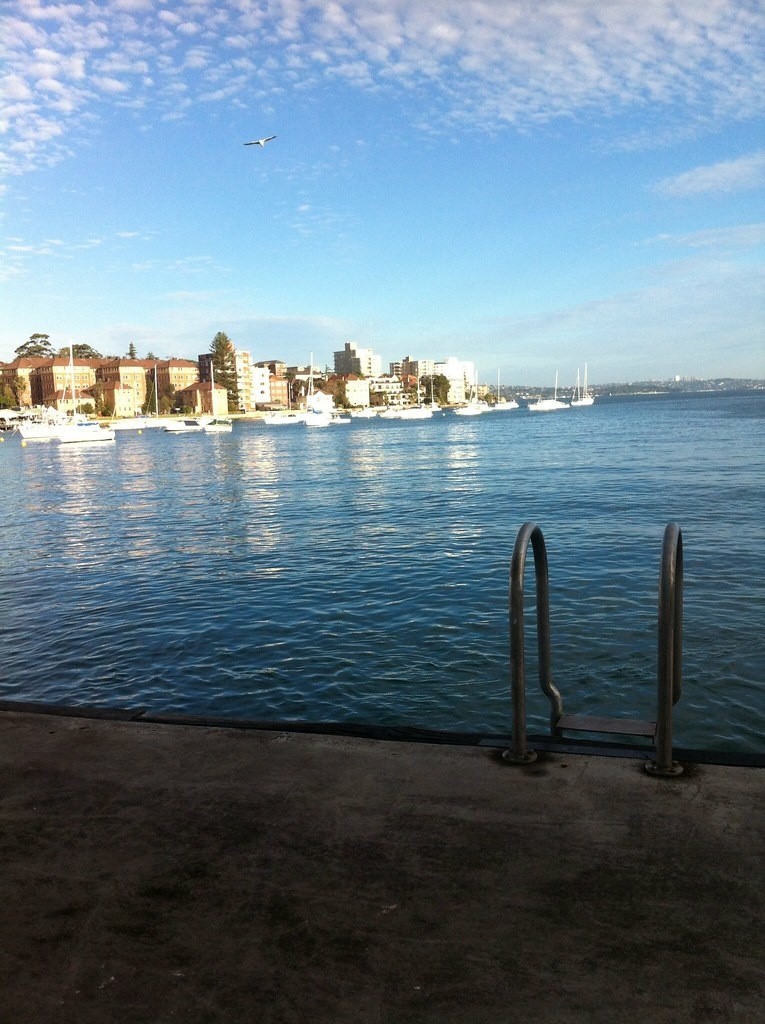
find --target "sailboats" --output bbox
[18,339,88,439]
[120,360,233,432]
[454,361,594,416]
[263,351,442,425]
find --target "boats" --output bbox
[56,422,115,442]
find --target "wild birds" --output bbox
[244,133,281,149]
